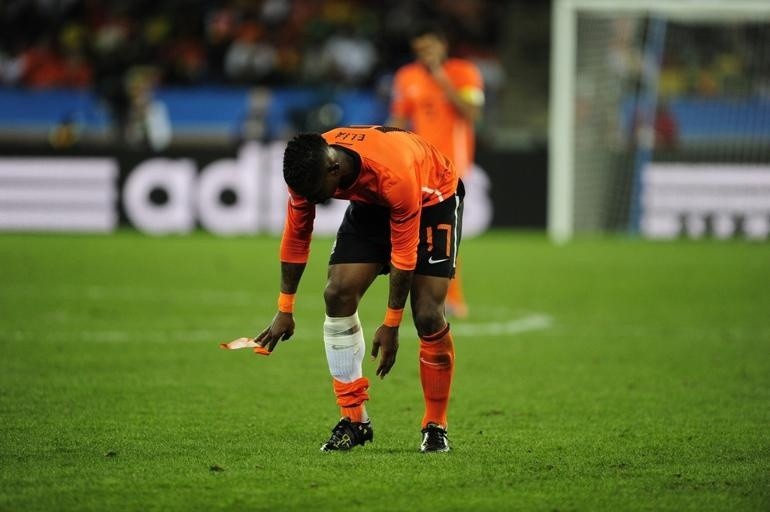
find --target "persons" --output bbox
[384,25,484,317]
[0,0,506,154]
[254,124,466,453]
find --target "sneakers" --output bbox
[320,416,374,450]
[420,422,450,452]
